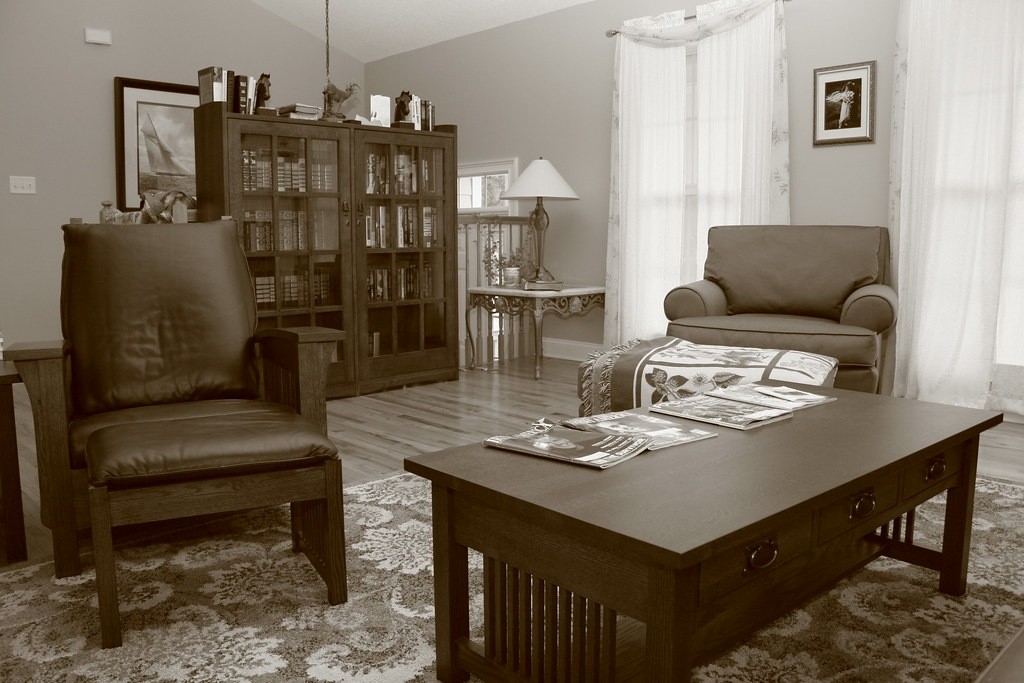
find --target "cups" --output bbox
[119,211,141,225]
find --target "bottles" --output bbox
[100,201,115,224]
[420,99,435,132]
[396,166,405,193]
[172,193,189,224]
[364,154,376,194]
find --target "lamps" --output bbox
[501,156,581,290]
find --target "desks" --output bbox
[465,287,605,380]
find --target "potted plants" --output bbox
[484,226,533,287]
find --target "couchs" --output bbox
[663,225,900,395]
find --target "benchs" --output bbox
[578,336,845,420]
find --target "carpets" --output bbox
[0,471,1024,683]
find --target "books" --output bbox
[198,65,256,115]
[560,410,718,450]
[650,382,837,431]
[367,154,438,301]
[520,279,562,291]
[482,416,655,469]
[242,146,336,310]
[406,95,435,131]
[277,103,322,121]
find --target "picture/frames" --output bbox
[114,76,200,213]
[813,60,878,145]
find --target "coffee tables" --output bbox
[402,381,1003,683]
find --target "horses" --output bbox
[394,90,412,122]
[254,72,271,111]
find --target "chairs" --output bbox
[2,220,348,648]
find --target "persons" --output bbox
[535,434,634,458]
[589,418,654,434]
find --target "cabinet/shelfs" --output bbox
[194,102,460,399]
